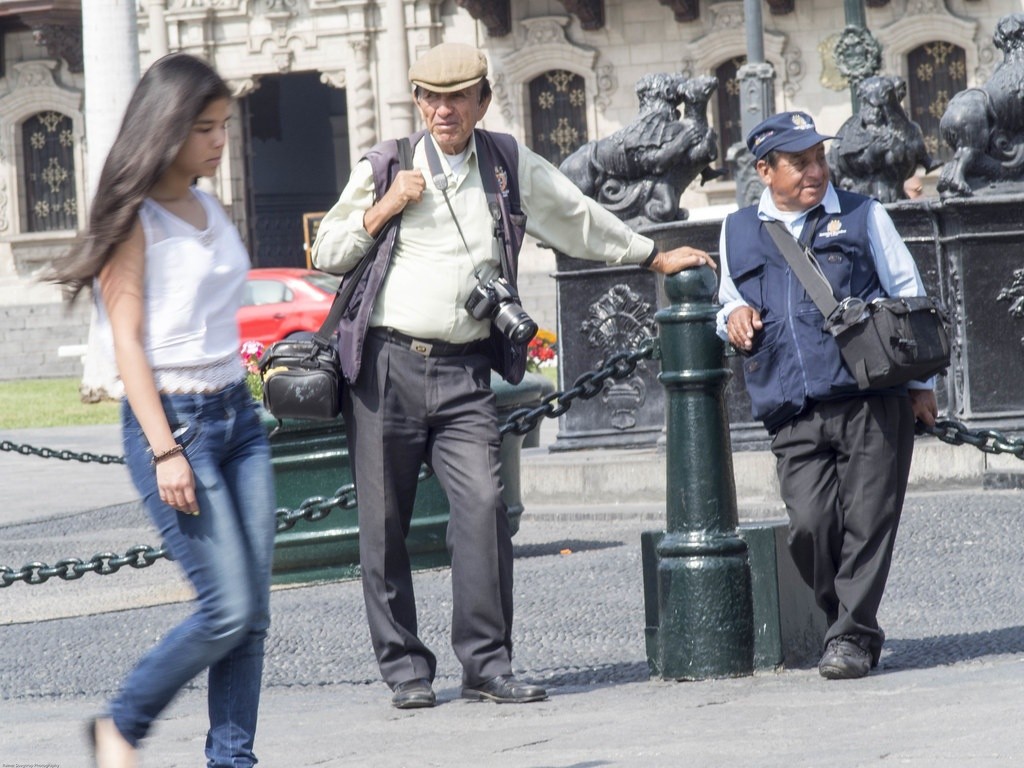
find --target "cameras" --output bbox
[464,277,539,347]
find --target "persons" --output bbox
[311,43,716,709]
[54,54,278,767]
[904,177,923,198]
[716,113,942,679]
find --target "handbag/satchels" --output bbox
[260,328,350,421]
[824,296,952,392]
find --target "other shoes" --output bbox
[818,640,872,679]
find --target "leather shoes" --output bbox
[392,678,436,707]
[462,674,548,703]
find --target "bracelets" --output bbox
[154,444,182,463]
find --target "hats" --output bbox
[408,41,488,93]
[746,111,843,162]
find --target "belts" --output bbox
[375,326,491,357]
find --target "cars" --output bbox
[238,267,343,346]
